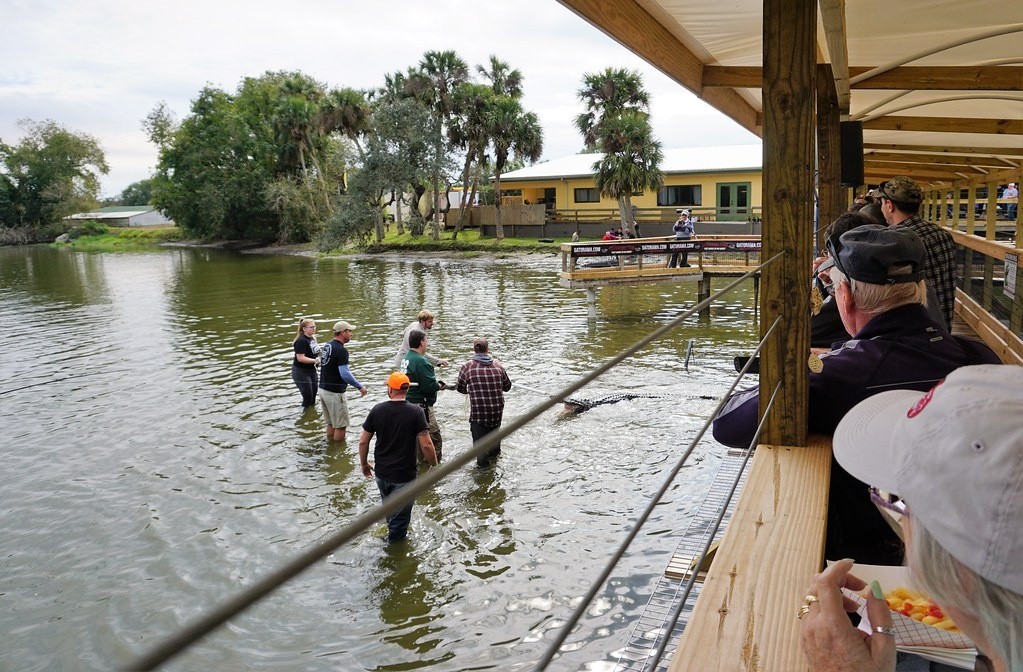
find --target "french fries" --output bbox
[861,586,961,634]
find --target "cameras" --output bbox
[679,221,684,224]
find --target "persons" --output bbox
[1003,183,1018,221]
[400,329,442,462]
[394,309,449,370]
[871,175,957,335]
[572,230,581,265]
[714,224,1001,566]
[799,363,1023,672]
[319,321,368,441]
[849,186,890,228]
[669,209,694,268]
[292,318,323,406]
[602,220,641,240]
[811,211,949,347]
[457,337,511,464]
[359,372,439,541]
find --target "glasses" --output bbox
[825,230,857,290]
[869,487,916,527]
[306,326,317,329]
[878,181,895,204]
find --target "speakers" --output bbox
[840,121,865,187]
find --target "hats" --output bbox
[474,338,488,350]
[833,364,1023,595]
[818,224,927,284]
[681,209,690,216]
[1009,182,1015,187]
[385,372,410,389]
[333,321,356,333]
[871,176,922,203]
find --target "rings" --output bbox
[796,612,801,619]
[805,595,819,604]
[799,606,809,618]
[874,627,897,636]
